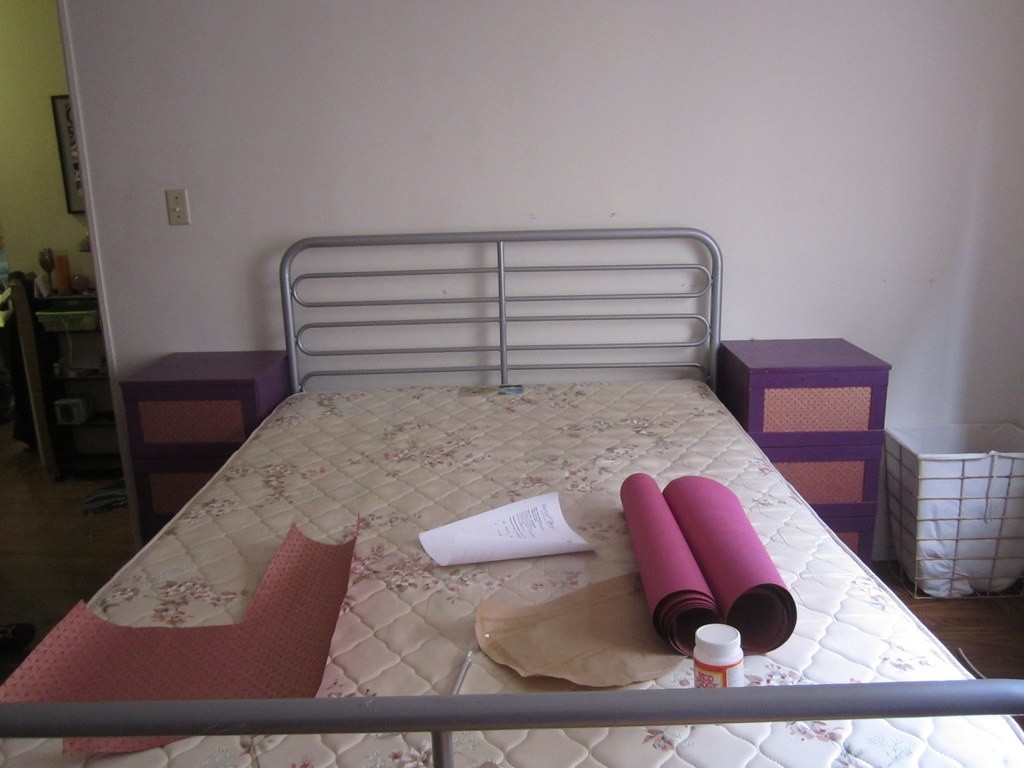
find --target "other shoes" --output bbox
[83,481,128,514]
[0,622,35,651]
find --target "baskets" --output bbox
[35,306,99,331]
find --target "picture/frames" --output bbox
[51,94,88,214]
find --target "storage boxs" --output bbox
[884,421,1024,603]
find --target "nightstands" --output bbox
[718,336,891,565]
[122,349,294,547]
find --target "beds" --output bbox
[0,227,1024,768]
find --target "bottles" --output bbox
[55,249,73,295]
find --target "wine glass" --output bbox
[39,248,55,295]
